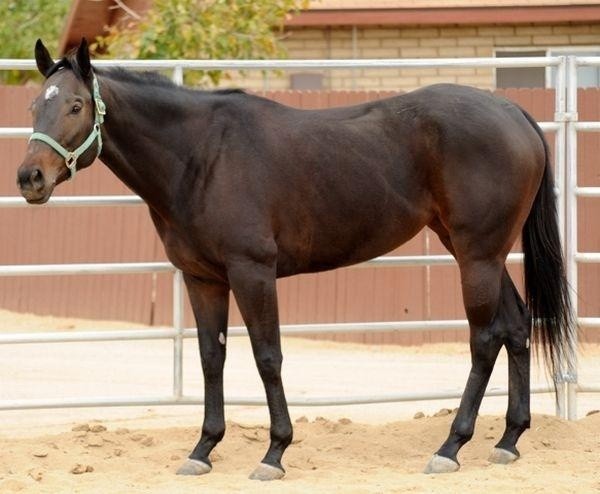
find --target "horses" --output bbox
[15,35,591,482]
